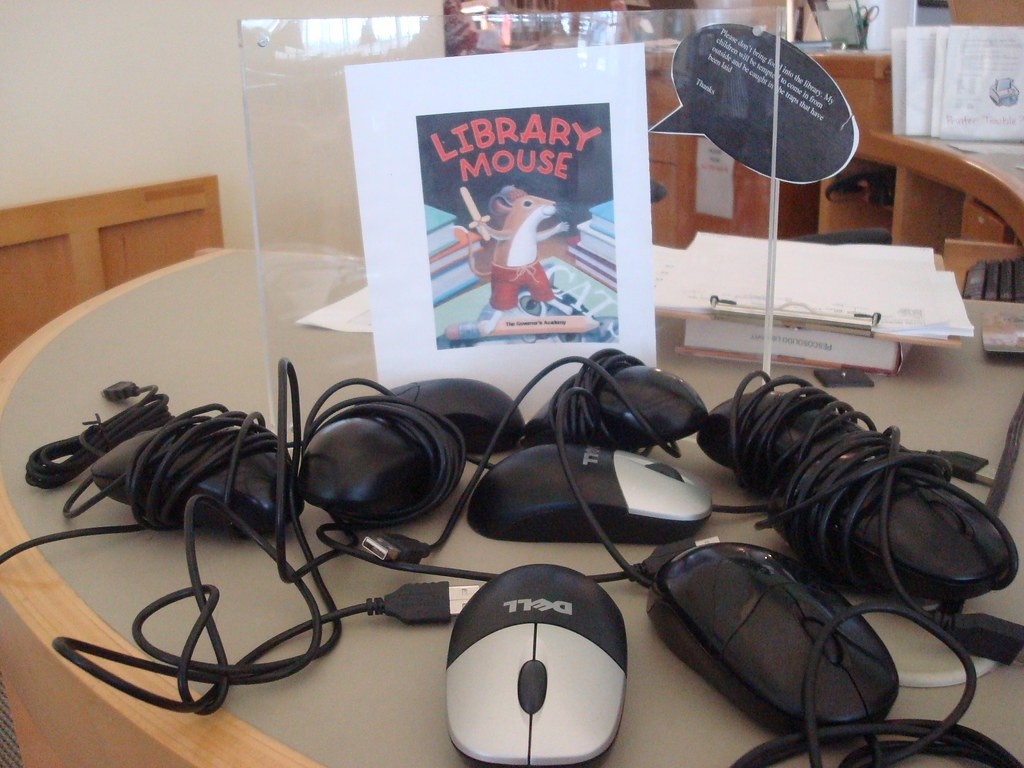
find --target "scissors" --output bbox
[856,5,880,50]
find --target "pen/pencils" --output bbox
[849,0,868,50]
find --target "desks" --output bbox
[0,248,1024,767]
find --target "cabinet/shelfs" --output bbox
[644,52,1023,258]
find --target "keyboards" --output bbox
[962,258,1024,304]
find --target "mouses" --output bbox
[91,350,1009,768]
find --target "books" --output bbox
[890,25,1024,145]
[961,254,1024,303]
[673,318,913,374]
